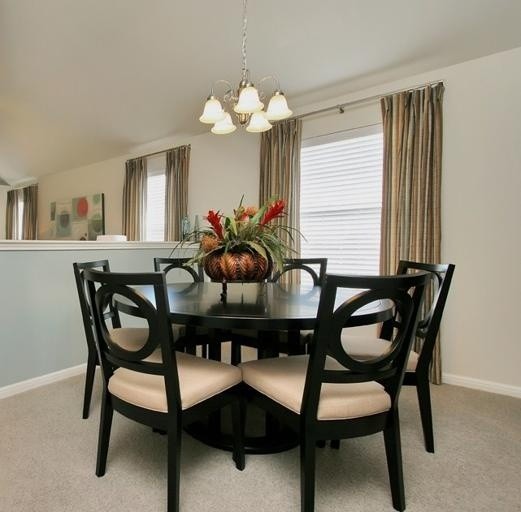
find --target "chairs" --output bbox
[73,260,182,419]
[238,272,431,512]
[154,256,328,365]
[84,268,245,512]
[308,259,455,454]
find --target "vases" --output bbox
[204,243,273,284]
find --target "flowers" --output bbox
[167,193,307,288]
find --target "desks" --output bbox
[113,280,394,456]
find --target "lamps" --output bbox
[198,0,297,137]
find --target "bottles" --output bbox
[195,215,202,242]
[183,216,191,241]
